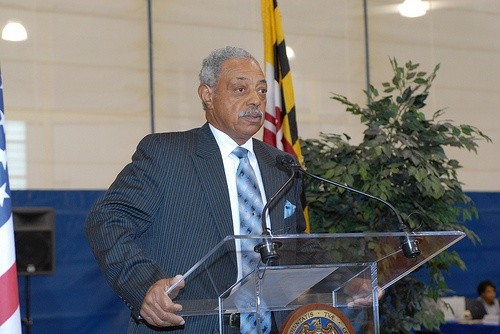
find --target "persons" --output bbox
[84,45,385,334]
[469,280,500,320]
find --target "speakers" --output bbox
[12,207,55,275]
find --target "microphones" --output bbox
[286,155,408,233]
[262,155,295,235]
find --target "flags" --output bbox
[260,0,310,236]
[0,66,22,334]
